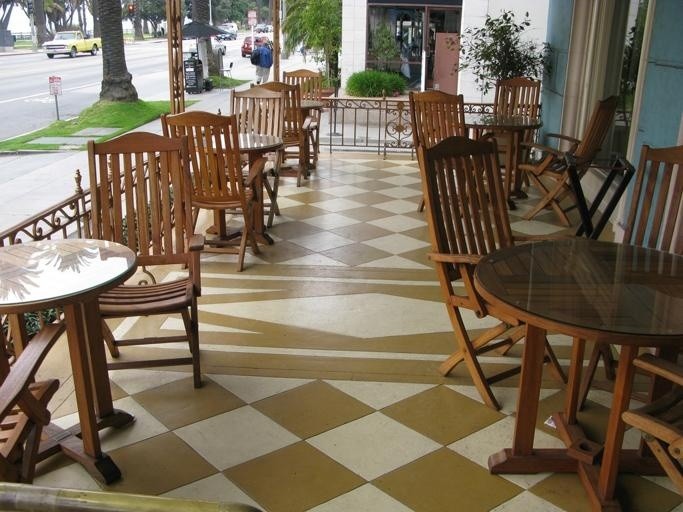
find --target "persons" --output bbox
[253,42,272,86]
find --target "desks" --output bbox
[257,99,324,176]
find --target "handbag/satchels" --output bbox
[251,49,260,65]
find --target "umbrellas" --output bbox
[182,20,230,64]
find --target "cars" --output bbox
[216,29,237,41]
[266,25,273,33]
[240,36,269,57]
[254,24,268,33]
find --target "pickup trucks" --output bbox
[41,31,101,58]
